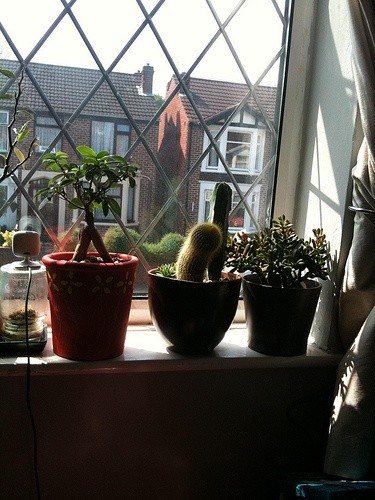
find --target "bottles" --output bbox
[1,263,47,343]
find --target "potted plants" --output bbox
[224,215,333,358]
[33,144,139,363]
[147,181,243,354]
